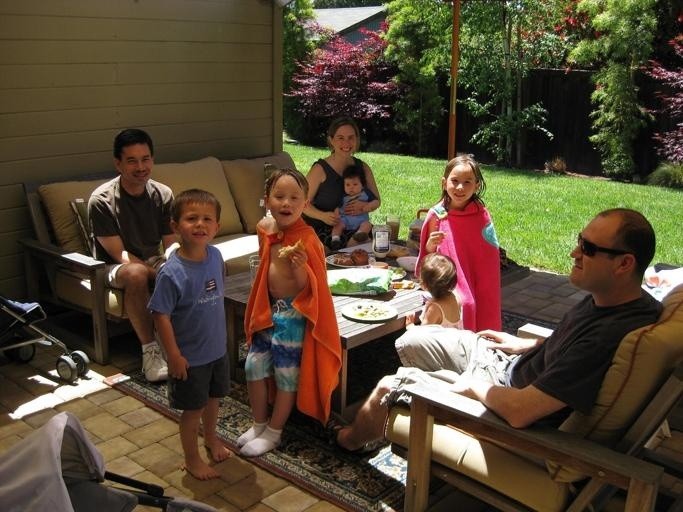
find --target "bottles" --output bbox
[371,224,392,262]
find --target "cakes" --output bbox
[278,239,305,259]
[387,247,409,258]
[333,249,368,265]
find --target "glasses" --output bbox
[577,233,629,257]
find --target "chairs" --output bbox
[383,282,683,512]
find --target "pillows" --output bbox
[37,179,111,253]
[69,197,97,260]
[149,155,244,244]
[220,151,297,234]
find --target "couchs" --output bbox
[18,151,297,366]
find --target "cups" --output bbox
[386,215,402,242]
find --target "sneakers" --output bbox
[352,231,368,242]
[331,240,342,248]
[308,413,365,463]
[141,345,168,382]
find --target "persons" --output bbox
[324,208,664,458]
[300,117,399,369]
[236,166,344,458]
[415,155,502,333]
[88,129,182,383]
[404,253,465,331]
[147,189,232,481]
[330,166,372,250]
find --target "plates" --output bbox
[386,266,407,280]
[342,299,398,323]
[326,253,375,268]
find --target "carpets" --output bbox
[105,309,559,512]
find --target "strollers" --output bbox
[0,295,90,383]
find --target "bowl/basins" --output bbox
[396,256,420,271]
